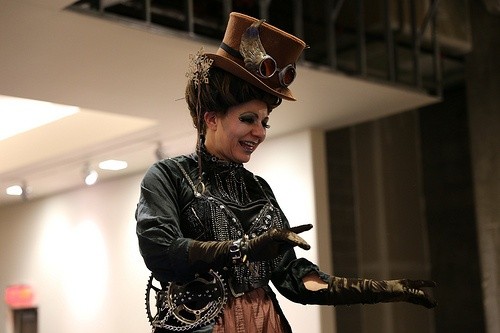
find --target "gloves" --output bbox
[189,221,313,266]
[331,275,441,310]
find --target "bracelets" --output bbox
[230,236,250,269]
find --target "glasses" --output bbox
[259,53,296,87]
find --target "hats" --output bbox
[202,12,306,102]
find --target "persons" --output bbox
[135,13,438,333]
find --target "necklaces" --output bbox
[197,141,252,206]
